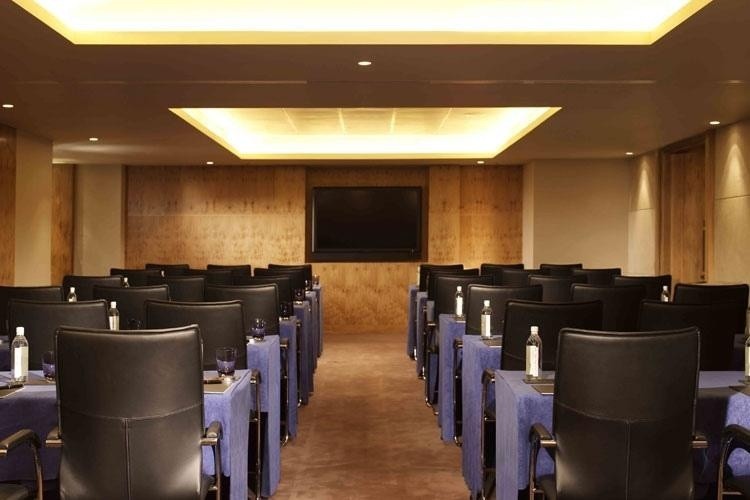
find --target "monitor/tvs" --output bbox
[311,185,424,254]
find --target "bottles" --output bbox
[453,285,464,320]
[479,299,497,341]
[10,325,29,382]
[66,287,78,303]
[108,300,120,330]
[660,285,670,304]
[524,324,545,385]
[122,277,131,288]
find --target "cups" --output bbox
[251,317,267,342]
[40,351,56,382]
[312,274,320,287]
[303,279,312,295]
[280,301,291,321]
[294,287,305,305]
[215,345,239,381]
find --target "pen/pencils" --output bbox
[0,385,23,389]
[203,380,222,384]
